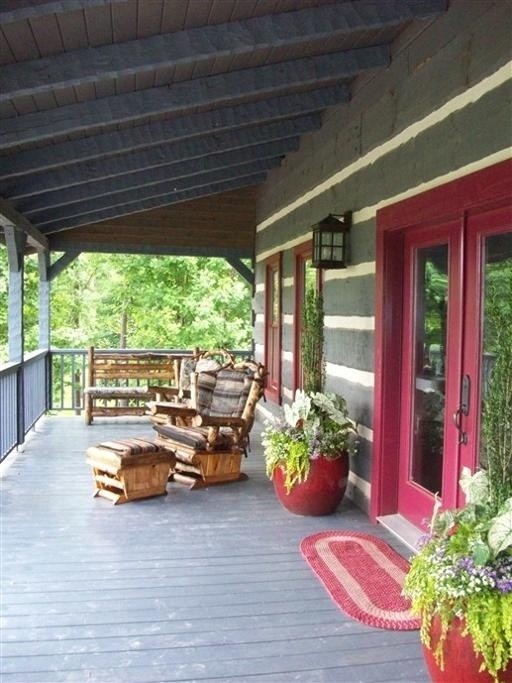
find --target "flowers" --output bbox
[255,386,365,496]
[395,463,512,683]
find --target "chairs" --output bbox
[143,346,235,440]
[148,359,267,492]
[149,350,208,402]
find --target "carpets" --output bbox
[294,526,428,634]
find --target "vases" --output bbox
[412,605,512,683]
[269,449,351,519]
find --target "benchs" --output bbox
[82,345,202,427]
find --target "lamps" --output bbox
[309,210,353,270]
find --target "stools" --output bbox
[83,435,176,507]
